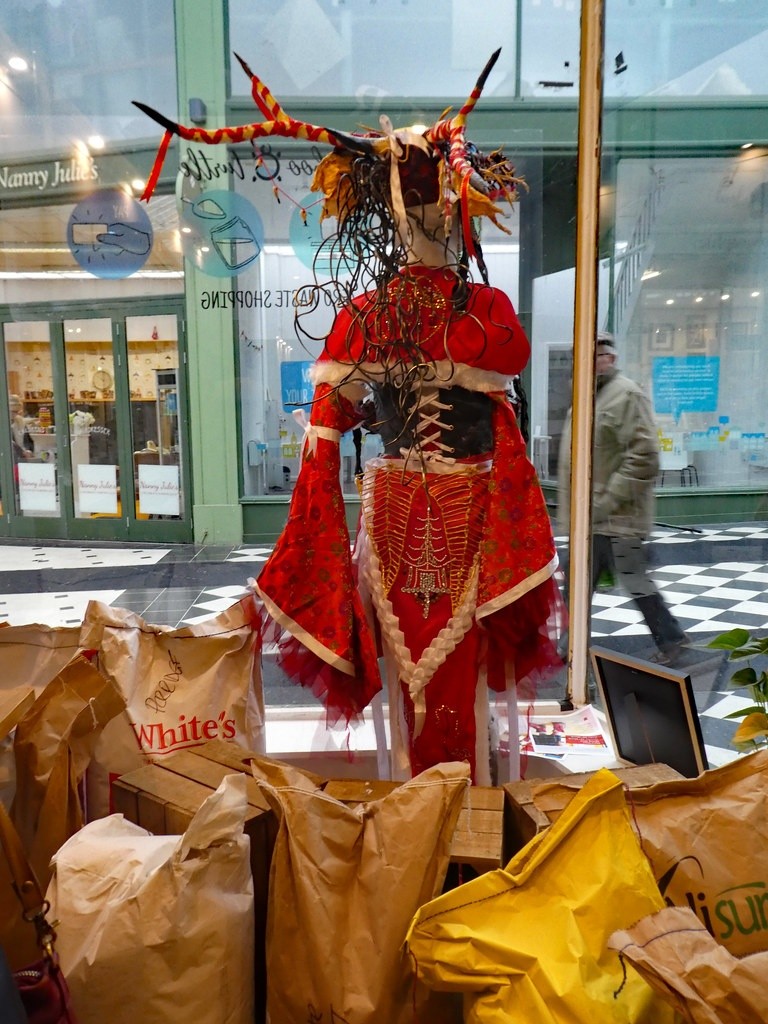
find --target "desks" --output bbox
[747,460,768,486]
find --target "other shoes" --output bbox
[647,633,693,667]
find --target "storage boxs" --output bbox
[110,740,329,930]
[502,762,688,850]
[322,780,506,889]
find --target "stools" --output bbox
[660,448,700,487]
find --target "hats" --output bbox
[598,331,615,345]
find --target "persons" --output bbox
[243,121,563,790]
[556,333,693,664]
[7,395,35,493]
